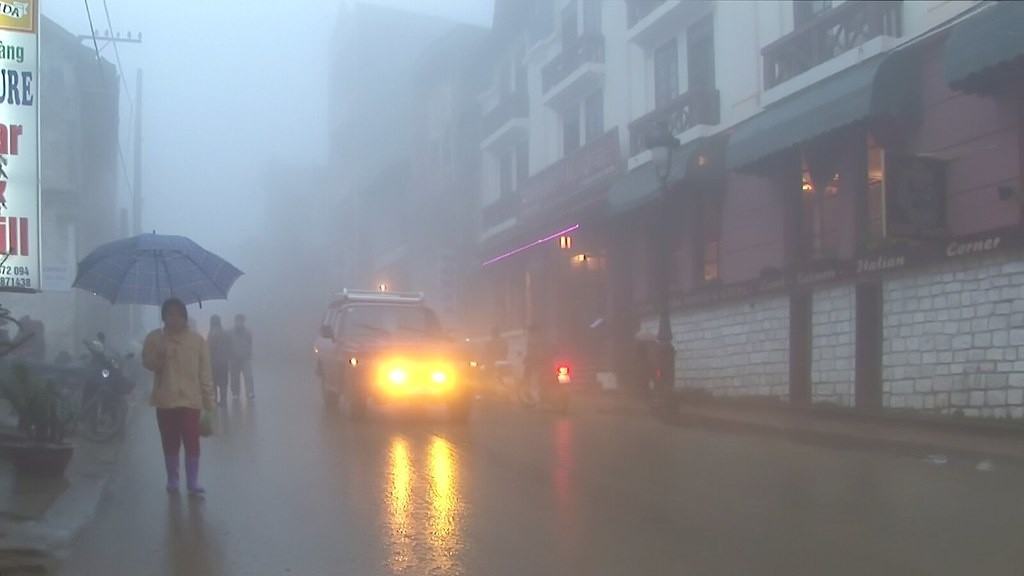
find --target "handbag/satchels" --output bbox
[199,410,213,437]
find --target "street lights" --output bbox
[641,119,682,385]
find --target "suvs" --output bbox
[311,286,472,427]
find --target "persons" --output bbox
[522,321,550,371]
[141,296,217,493]
[206,313,255,406]
[481,327,510,388]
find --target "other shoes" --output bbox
[217,400,226,407]
[233,391,240,400]
[247,391,255,399]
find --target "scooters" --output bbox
[514,350,577,419]
[69,331,136,446]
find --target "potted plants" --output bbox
[0,362,79,476]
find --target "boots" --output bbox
[184,453,204,492]
[162,447,181,492]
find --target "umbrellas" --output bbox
[69,229,247,344]
